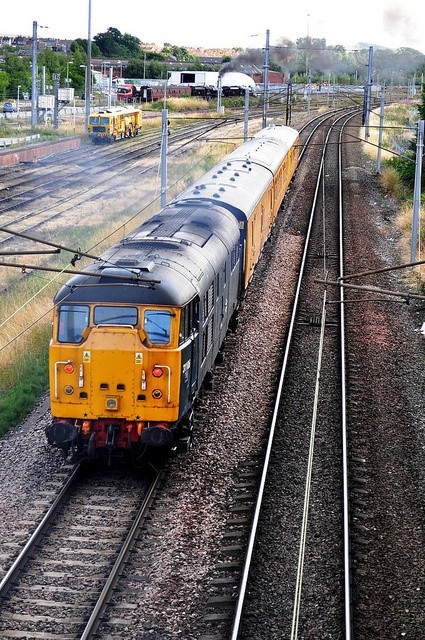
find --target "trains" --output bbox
[88,108,141,142]
[47,123,299,467]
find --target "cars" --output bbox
[3,103,13,113]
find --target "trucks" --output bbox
[118,84,191,101]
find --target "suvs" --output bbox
[190,86,217,96]
[223,86,245,96]
